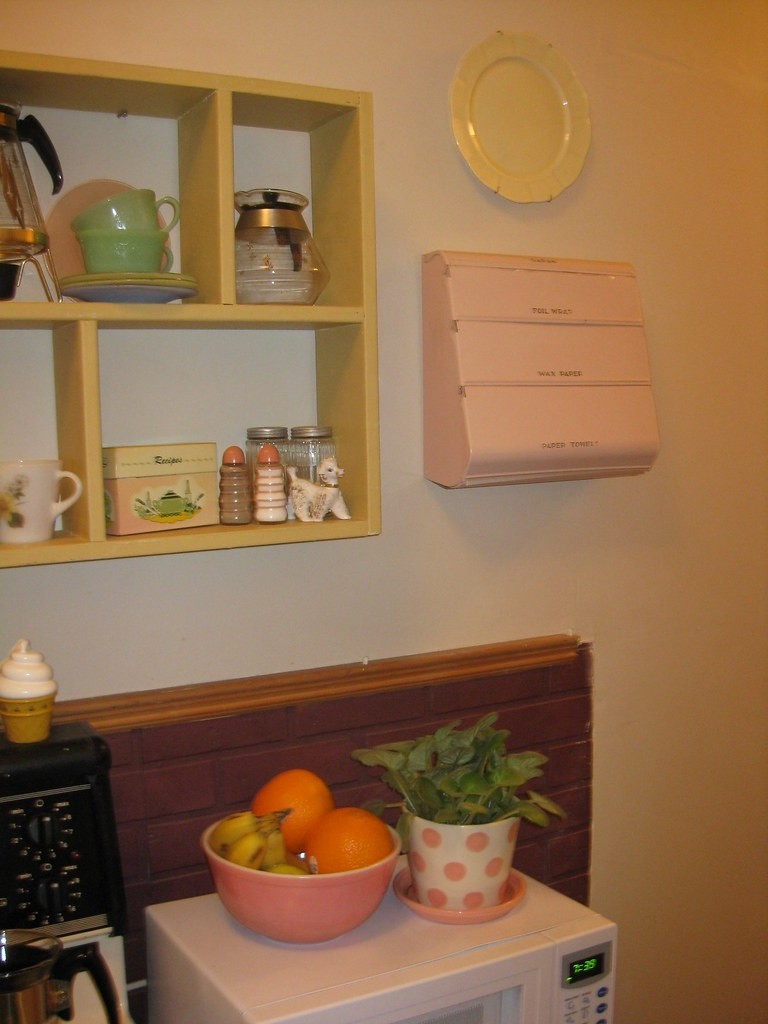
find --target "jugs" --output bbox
[0,95,64,262]
[0,928,125,1024]
[233,189,331,306]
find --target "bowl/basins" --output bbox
[199,817,402,945]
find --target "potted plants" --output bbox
[352,710,568,922]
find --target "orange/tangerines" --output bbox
[252,768,395,874]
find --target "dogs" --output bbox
[287,457,351,522]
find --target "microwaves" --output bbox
[145,857,620,1024]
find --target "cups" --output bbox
[0,458,83,545]
[70,189,180,233]
[0,689,58,744]
[75,230,174,273]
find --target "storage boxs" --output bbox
[101,442,219,536]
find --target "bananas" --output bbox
[209,808,310,875]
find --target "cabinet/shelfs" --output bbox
[0,50,381,568]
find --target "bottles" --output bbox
[290,426,337,518]
[253,441,288,524]
[246,424,290,486]
[219,446,252,526]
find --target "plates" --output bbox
[58,273,199,304]
[43,178,171,303]
[450,30,594,204]
